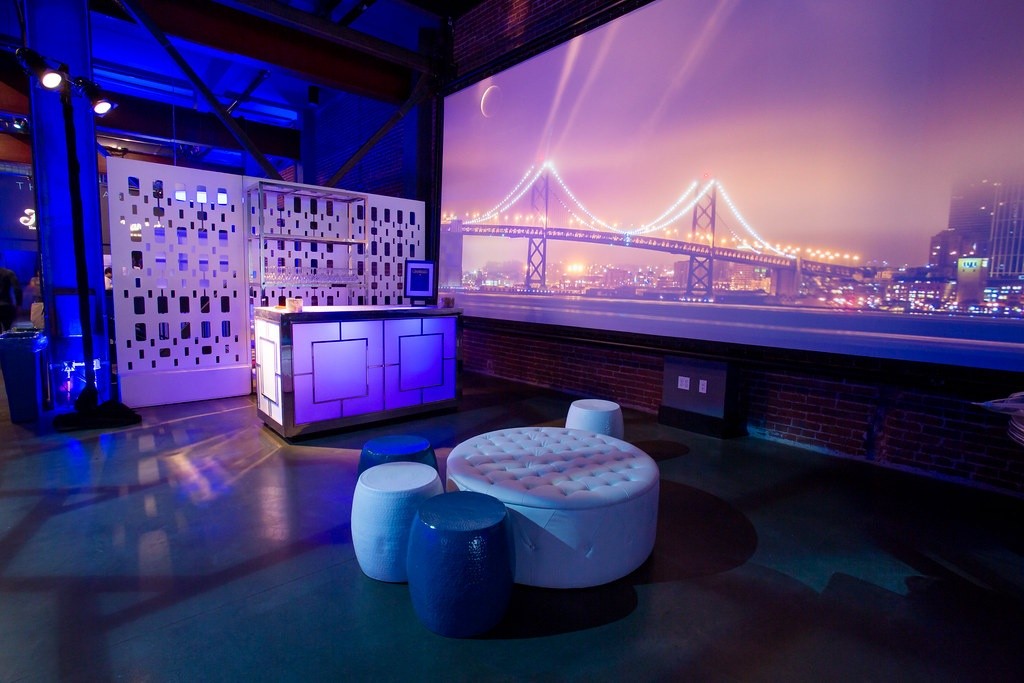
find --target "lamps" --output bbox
[75,77,113,113]
[17,47,63,89]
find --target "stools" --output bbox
[355,435,438,476]
[405,490,516,637]
[565,398,623,442]
[352,461,445,583]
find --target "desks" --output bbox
[449,425,659,590]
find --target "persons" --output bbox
[0,267,23,334]
[104,267,113,290]
[132,253,142,267]
[30,269,42,302]
